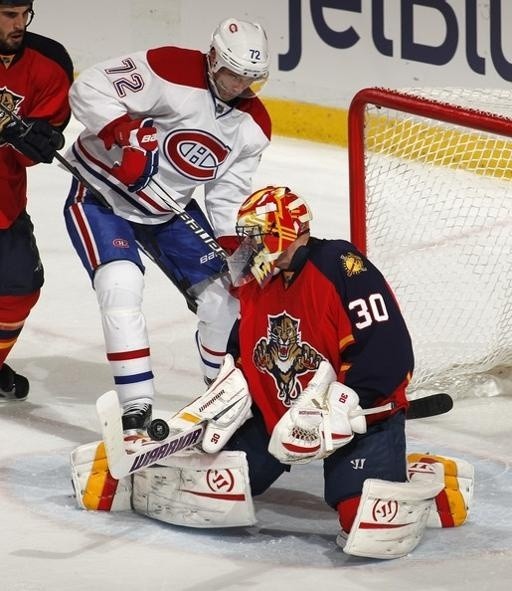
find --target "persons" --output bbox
[61,15,273,432]
[68,188,477,560]
[0,0,73,398]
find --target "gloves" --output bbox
[1,116,65,166]
[111,114,162,195]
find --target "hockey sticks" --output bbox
[95,390,254,479]
[148,178,453,419]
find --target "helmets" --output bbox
[206,17,271,104]
[234,185,312,292]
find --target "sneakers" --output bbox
[0,361,30,402]
[121,400,153,431]
